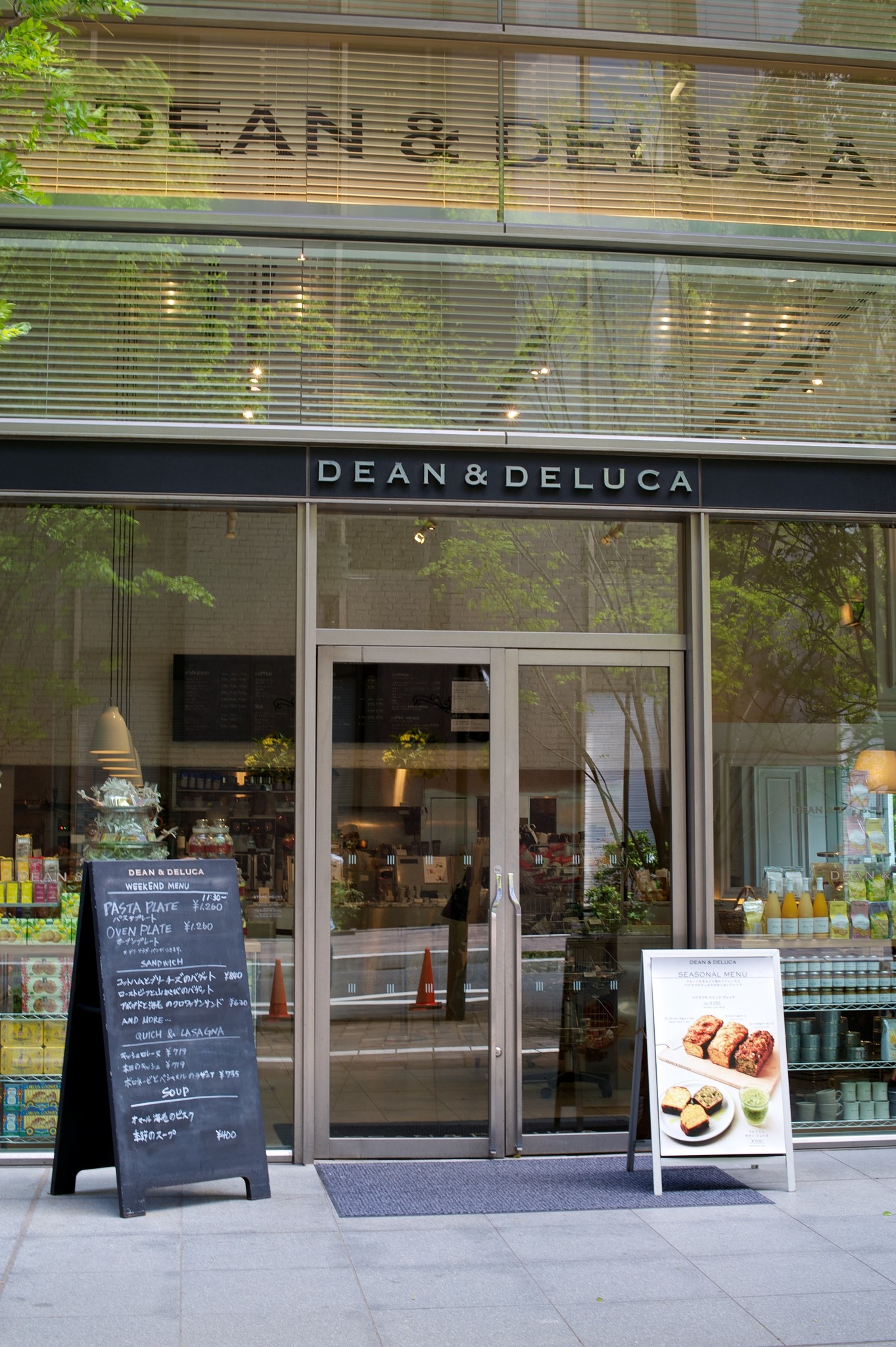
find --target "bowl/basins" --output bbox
[785,1009,881,1064]
[356,840,368,848]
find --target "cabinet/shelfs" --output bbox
[714,938,896,1149]
[87,805,168,850]
[172,773,294,811]
[521,845,628,1099]
[0,945,75,1166]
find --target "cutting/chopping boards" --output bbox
[658,1037,781,1096]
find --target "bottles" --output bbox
[782,878,798,940]
[765,878,782,939]
[248,840,256,854]
[206,818,235,860]
[798,877,813,939]
[813,877,829,939]
[188,819,210,859]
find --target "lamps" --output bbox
[838,598,865,628]
[415,515,438,544]
[225,511,239,538]
[90,506,143,788]
[602,521,628,546]
[854,750,896,817]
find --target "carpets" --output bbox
[274,1116,648,1146]
[315,1155,775,1218]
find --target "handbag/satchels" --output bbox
[440,867,471,921]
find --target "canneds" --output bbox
[780,954,896,1005]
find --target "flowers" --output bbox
[382,727,436,774]
[244,731,296,782]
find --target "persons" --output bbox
[338,825,378,904]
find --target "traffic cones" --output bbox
[408,948,444,1011]
[261,957,292,1022]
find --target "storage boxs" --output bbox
[3,1083,61,1137]
[0,893,81,946]
[0,1020,68,1075]
[21,956,74,1013]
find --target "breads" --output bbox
[661,1086,691,1115]
[707,1021,749,1070]
[693,1085,723,1115]
[734,1030,775,1078]
[680,1104,710,1136]
[683,1014,723,1059]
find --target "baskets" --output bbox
[717,885,759,934]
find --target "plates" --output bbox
[659,1080,736,1139]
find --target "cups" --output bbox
[432,839,441,856]
[422,841,429,856]
[739,1083,770,1125]
[246,904,278,938]
[381,844,390,858]
[411,841,421,856]
[790,1076,896,1122]
[176,772,295,791]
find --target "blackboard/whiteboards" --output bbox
[54,858,269,1191]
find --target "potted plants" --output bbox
[583,830,660,926]
[330,834,364,930]
